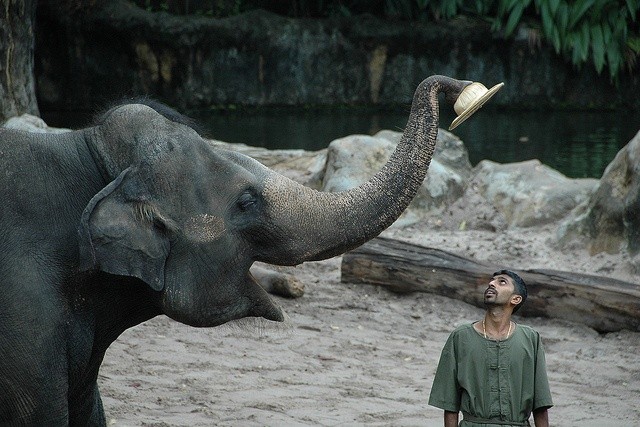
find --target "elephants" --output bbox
[0,75,472,427]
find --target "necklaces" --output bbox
[482,319,512,340]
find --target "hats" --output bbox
[448,81,504,132]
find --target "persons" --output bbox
[427,269,553,427]
